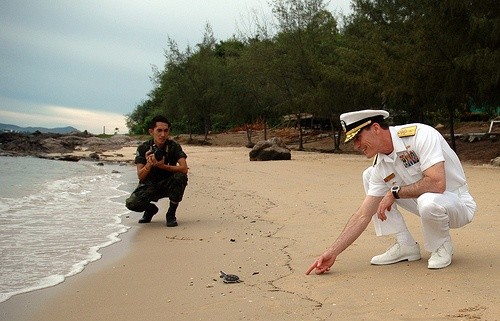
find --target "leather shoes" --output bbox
[370,241,420,264]
[427,238,454,268]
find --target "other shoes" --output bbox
[166,213,177,226]
[138,203,159,223]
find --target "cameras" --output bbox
[149,144,163,162]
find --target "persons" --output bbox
[126,116,189,227]
[306,109,477,276]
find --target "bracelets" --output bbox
[144,166,150,172]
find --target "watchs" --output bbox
[391,185,401,199]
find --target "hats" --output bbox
[340,109,389,144]
